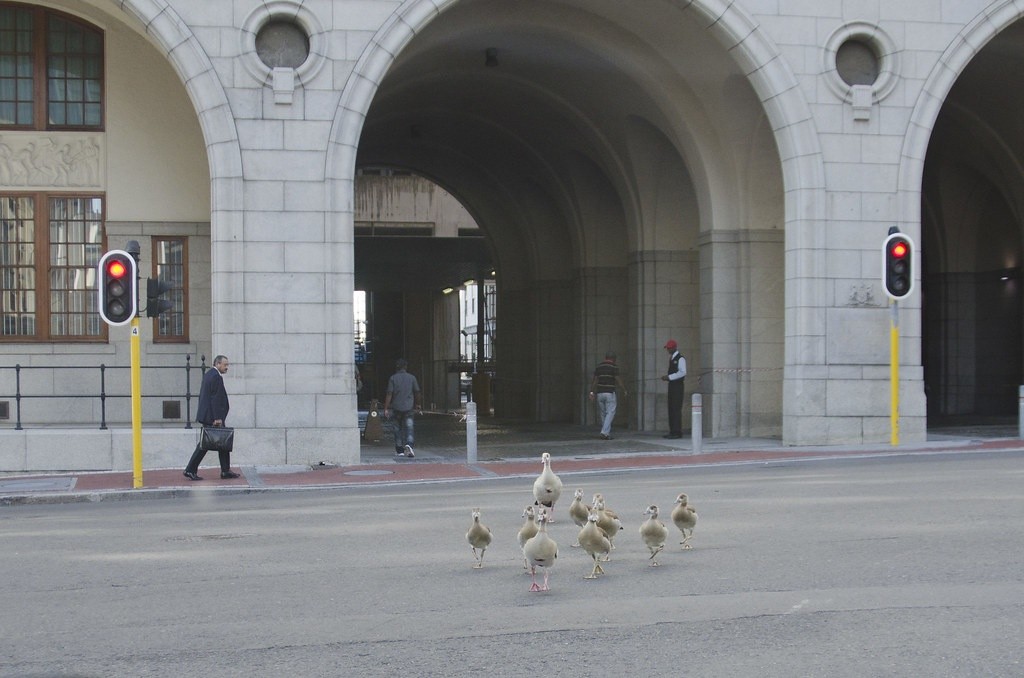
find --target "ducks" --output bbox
[578,511,610,578]
[639,504,668,566]
[671,493,698,544]
[588,493,623,551]
[465,507,494,569]
[532,451,562,523]
[516,505,538,574]
[569,487,592,527]
[524,508,559,592]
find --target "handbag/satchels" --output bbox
[199,422,234,452]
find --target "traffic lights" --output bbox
[98,250,138,327]
[882,232,914,302]
[146,276,174,318]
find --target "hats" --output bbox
[664,340,677,349]
[606,351,616,357]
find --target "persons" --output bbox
[385,359,421,457]
[589,350,628,440]
[661,340,687,439]
[183,355,240,481]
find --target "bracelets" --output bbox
[590,392,593,394]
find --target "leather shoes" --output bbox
[182,470,204,480]
[221,470,241,478]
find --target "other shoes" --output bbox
[599,433,612,439]
[398,452,405,457]
[405,445,415,457]
[663,433,682,439]
